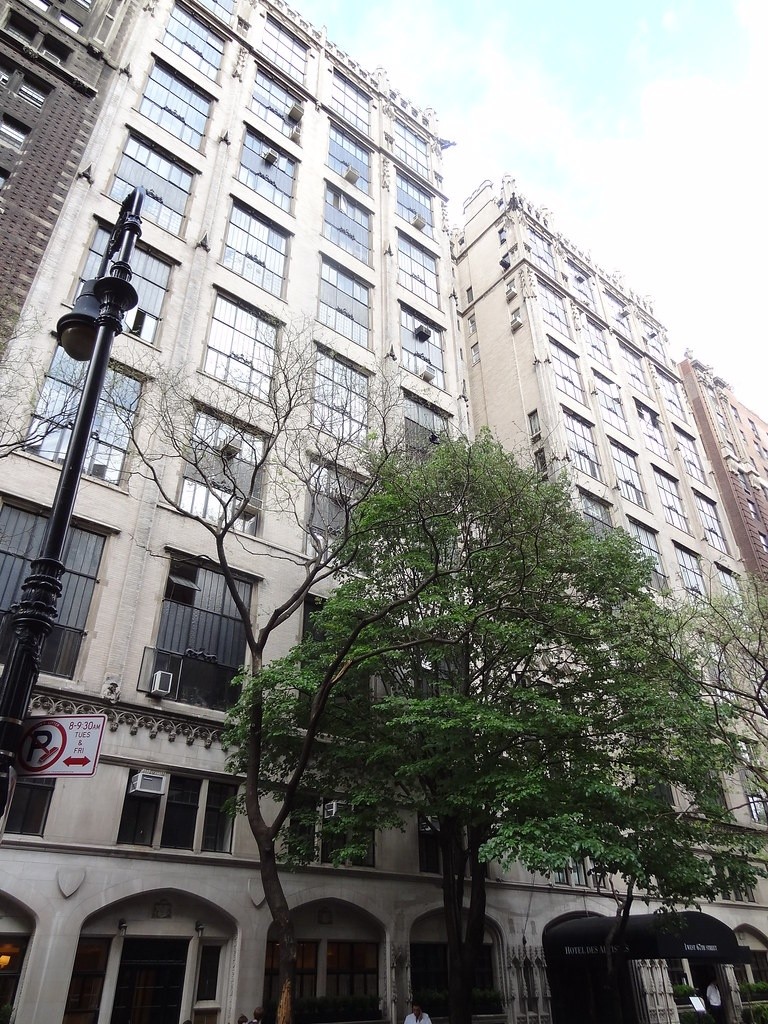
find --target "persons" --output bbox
[705,977,727,1024]
[403,1000,432,1024]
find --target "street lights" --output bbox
[1,184,145,807]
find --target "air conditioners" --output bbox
[225,437,241,453]
[266,147,278,163]
[152,672,173,696]
[289,103,304,121]
[411,214,427,229]
[510,317,522,331]
[129,772,165,800]
[42,50,60,65]
[333,484,352,500]
[416,326,431,341]
[291,126,301,138]
[240,496,263,516]
[620,309,631,317]
[648,329,656,336]
[419,364,436,382]
[577,273,584,281]
[343,166,359,184]
[506,288,517,300]
[420,815,439,831]
[324,800,353,819]
